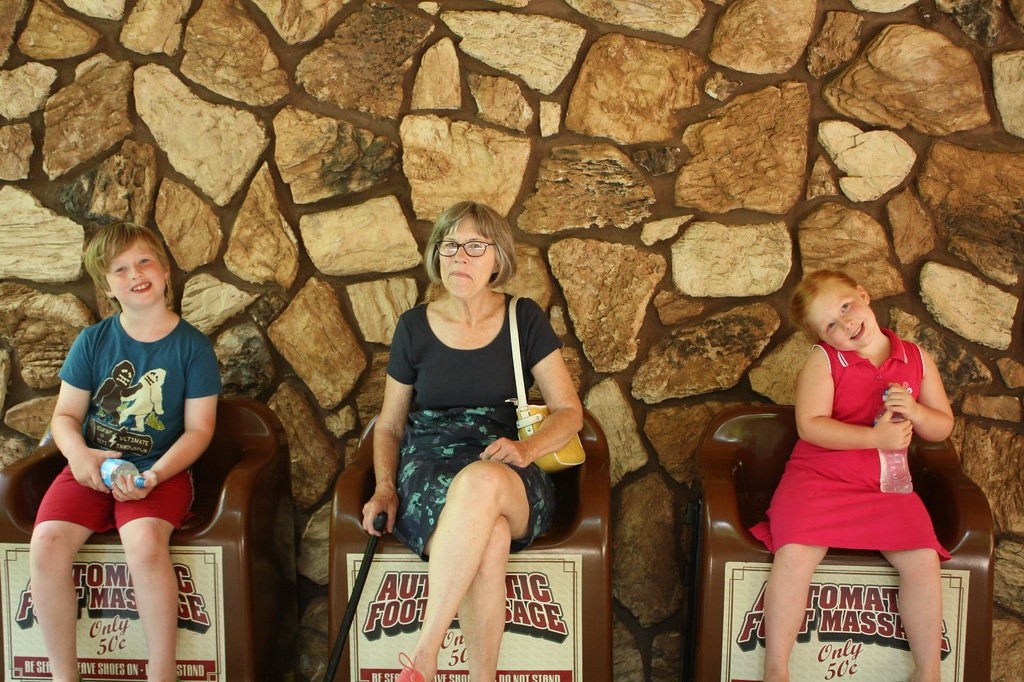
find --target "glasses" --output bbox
[435,240,496,257]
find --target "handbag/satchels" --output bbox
[509,295,586,474]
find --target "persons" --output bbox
[748,268,955,682]
[28,221,225,682]
[362,200,583,682]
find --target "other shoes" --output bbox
[395,652,434,682]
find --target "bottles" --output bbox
[872,390,914,494]
[98,457,146,490]
